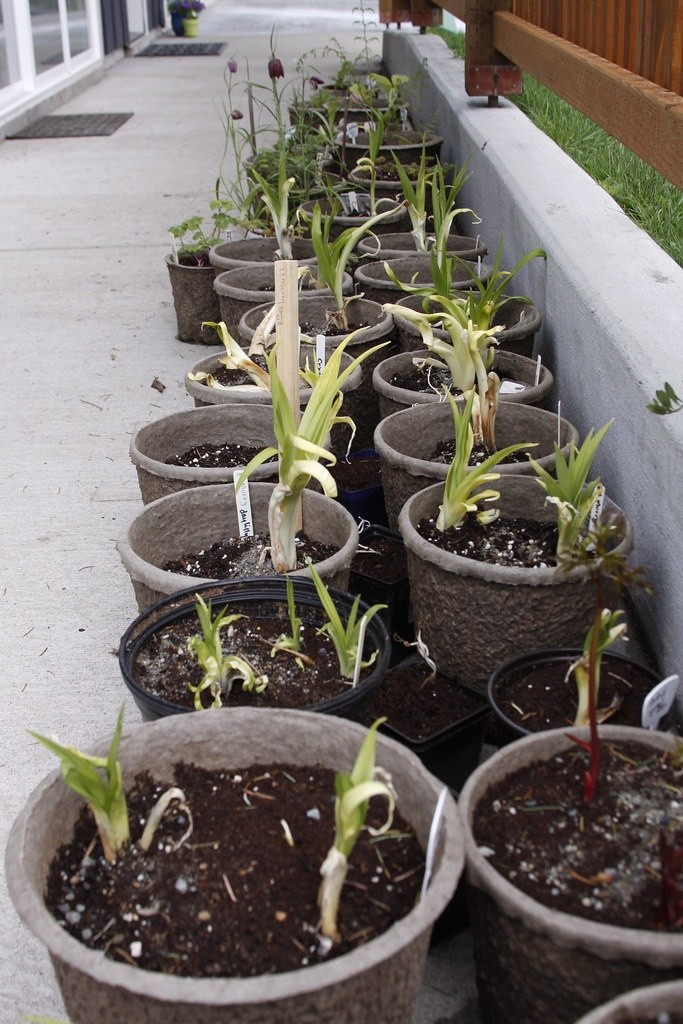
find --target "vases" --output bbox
[182,18,199,37]
[172,16,183,36]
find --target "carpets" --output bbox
[134,42,227,57]
[4,113,132,140]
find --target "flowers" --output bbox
[175,0,205,19]
[166,0,181,16]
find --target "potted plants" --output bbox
[0,0,682,1024]
